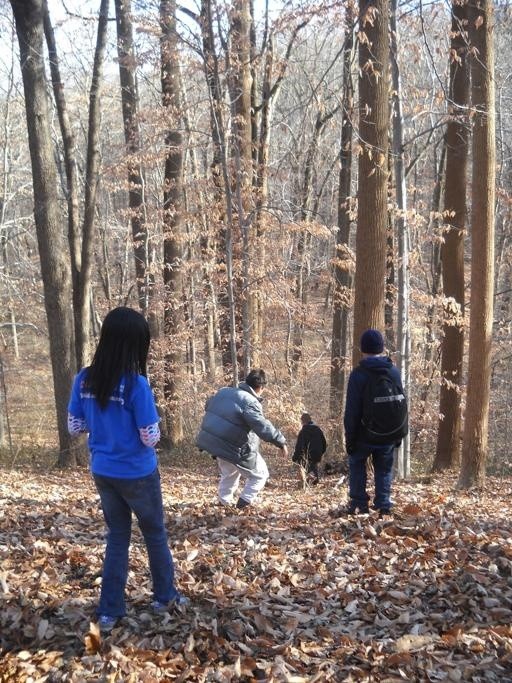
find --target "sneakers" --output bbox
[236,498,254,508]
[344,502,369,514]
[96,615,121,633]
[153,596,191,613]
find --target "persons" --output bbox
[336,327,410,516]
[291,413,329,488]
[192,367,290,510]
[67,304,192,632]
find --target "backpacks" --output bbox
[355,366,409,446]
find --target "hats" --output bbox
[360,330,384,354]
